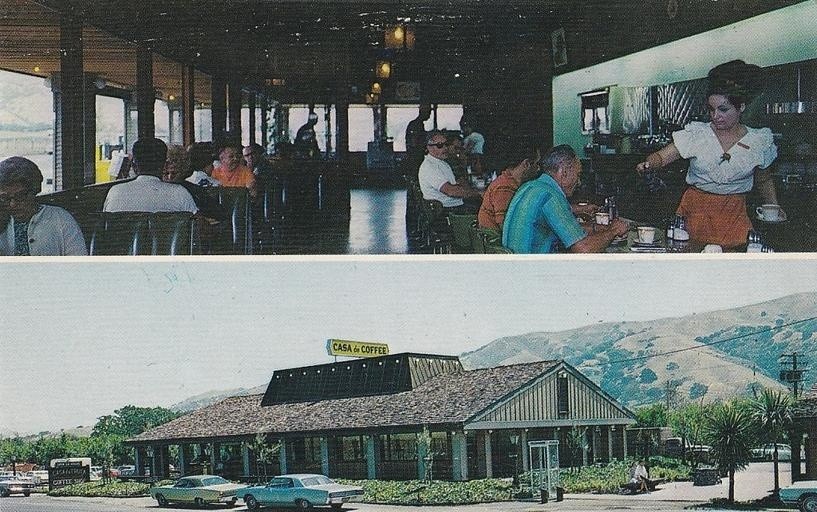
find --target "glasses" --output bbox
[427,141,449,148]
[453,143,465,151]
[0,189,33,202]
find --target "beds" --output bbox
[607,217,731,253]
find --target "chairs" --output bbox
[468,219,500,254]
[449,208,478,254]
[484,235,514,254]
[404,174,452,254]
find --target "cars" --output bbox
[752,441,794,463]
[1,463,174,499]
[238,474,364,510]
[152,475,239,507]
[779,480,817,512]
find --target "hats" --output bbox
[708,59,766,104]
[418,103,435,111]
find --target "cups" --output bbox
[756,204,781,221]
[594,212,611,227]
[475,179,484,189]
[700,245,723,253]
[637,226,657,244]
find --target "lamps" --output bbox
[376,53,395,80]
[365,78,382,108]
[384,22,417,52]
[264,68,287,86]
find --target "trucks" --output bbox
[665,437,685,460]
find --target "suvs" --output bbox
[685,446,716,467]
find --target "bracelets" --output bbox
[590,221,596,232]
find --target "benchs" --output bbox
[71,211,206,255]
[202,186,258,255]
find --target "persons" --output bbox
[501,142,631,253]
[629,461,652,494]
[406,103,486,238]
[478,139,541,229]
[0,156,89,256]
[102,136,200,215]
[634,58,787,247]
[164,111,321,203]
